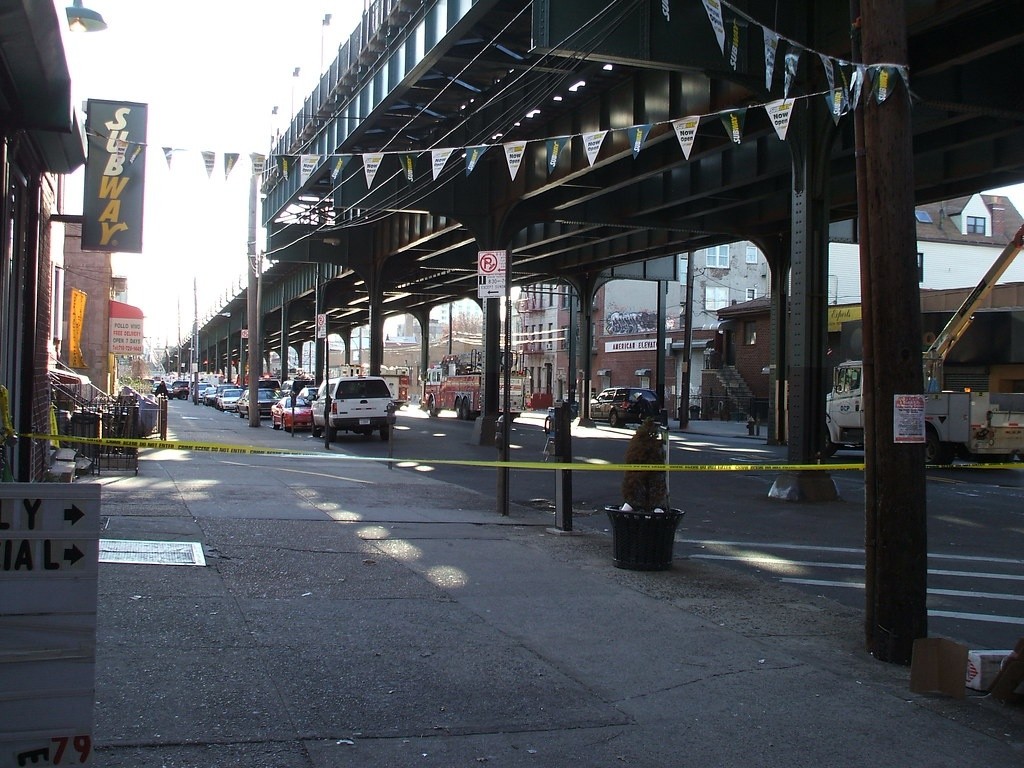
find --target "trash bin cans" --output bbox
[411,393,420,405]
[103,410,127,455]
[677,407,681,421]
[71,409,101,458]
[689,405,700,420]
[547,407,555,431]
[52,409,71,448]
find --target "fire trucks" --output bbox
[337,363,411,409]
[421,348,525,421]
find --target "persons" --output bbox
[155,381,168,398]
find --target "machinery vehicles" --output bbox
[917,222,1024,393]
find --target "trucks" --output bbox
[818,361,1024,467]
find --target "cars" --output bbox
[151,373,318,433]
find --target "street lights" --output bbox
[187,312,231,403]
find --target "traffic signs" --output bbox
[0,537,99,573]
[0,483,99,537]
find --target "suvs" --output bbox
[311,376,398,443]
[590,387,663,429]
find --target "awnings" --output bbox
[635,370,651,377]
[110,300,143,354]
[597,370,611,377]
